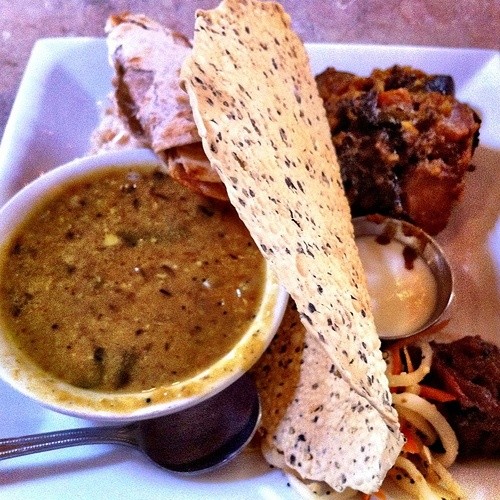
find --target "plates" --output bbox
[0,37,500,499]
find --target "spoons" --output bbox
[0,375,261,476]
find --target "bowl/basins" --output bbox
[0,147,289,422]
[347,214,454,340]
